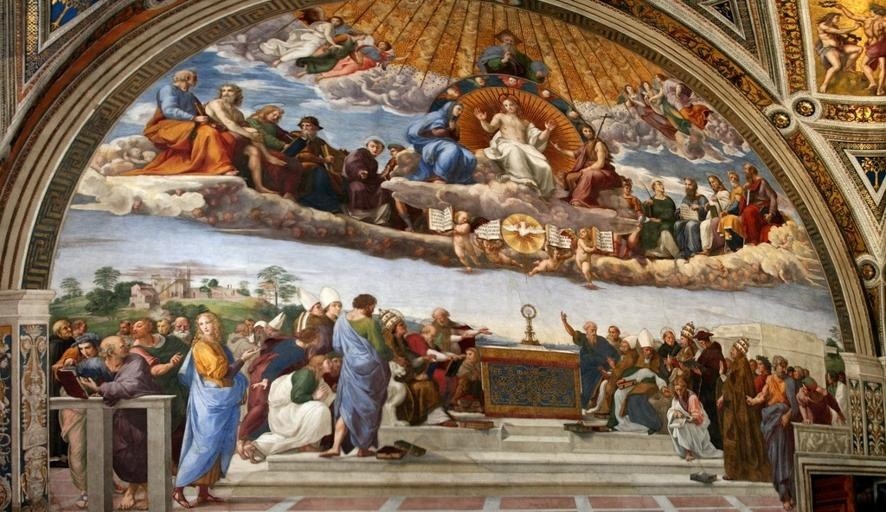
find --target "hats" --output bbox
[731,336,751,356]
[694,329,715,341]
[377,307,406,334]
[365,135,406,152]
[494,28,523,46]
[320,286,343,310]
[298,286,321,313]
[660,326,676,343]
[71,332,101,348]
[622,327,656,350]
[680,321,697,342]
[252,311,287,331]
[296,115,324,132]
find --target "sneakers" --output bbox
[235,437,263,465]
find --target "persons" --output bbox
[816,13,863,93]
[406,102,476,184]
[823,3,886,95]
[473,94,569,199]
[453,211,481,271]
[479,29,546,84]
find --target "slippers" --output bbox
[74,493,91,509]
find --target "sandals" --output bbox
[172,489,227,509]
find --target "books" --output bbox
[428,205,455,234]
